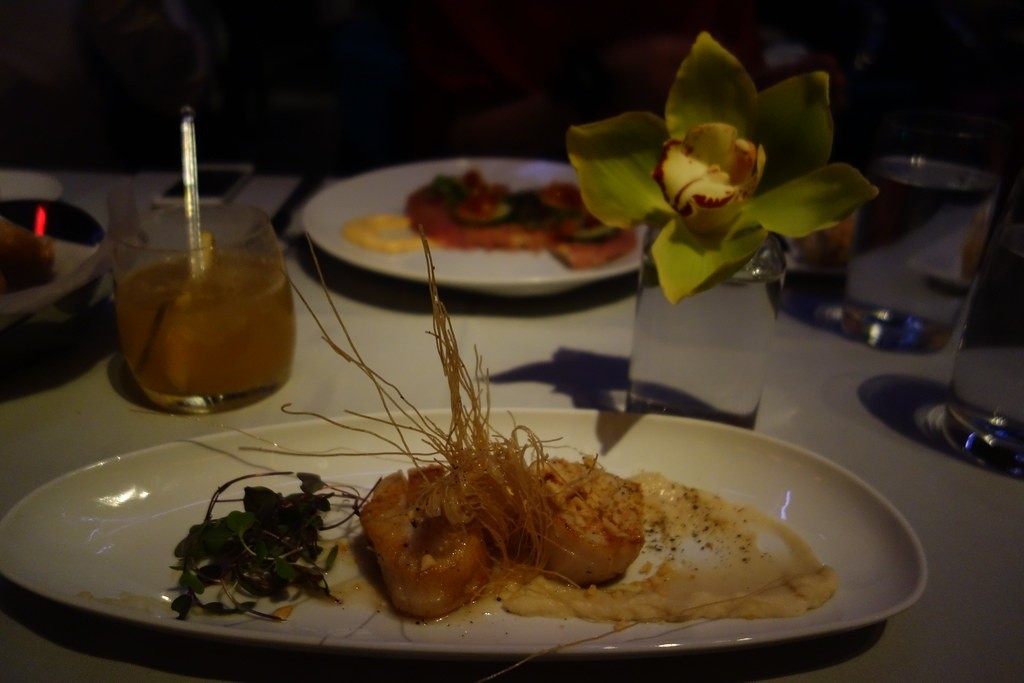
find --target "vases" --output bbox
[624,226,789,438]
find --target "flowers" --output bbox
[565,32,882,307]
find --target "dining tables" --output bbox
[0,170,1024,683]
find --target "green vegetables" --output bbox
[170,345,382,621]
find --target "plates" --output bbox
[0,407,929,664]
[0,170,62,202]
[301,156,641,301]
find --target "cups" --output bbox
[107,197,296,417]
[842,109,1024,361]
[943,166,1024,481]
